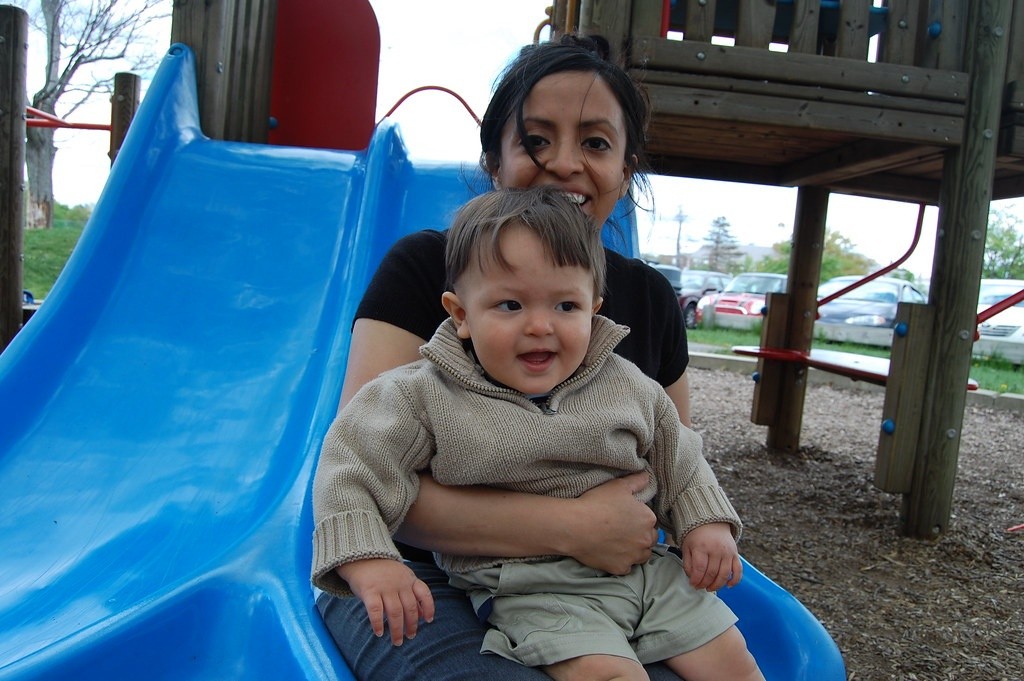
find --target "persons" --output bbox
[309,188,766,681]
[311,33,690,681]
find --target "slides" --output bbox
[0,40,846,681]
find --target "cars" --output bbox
[647,261,735,330]
[695,271,789,331]
[811,275,929,348]
[970,278,1024,368]
